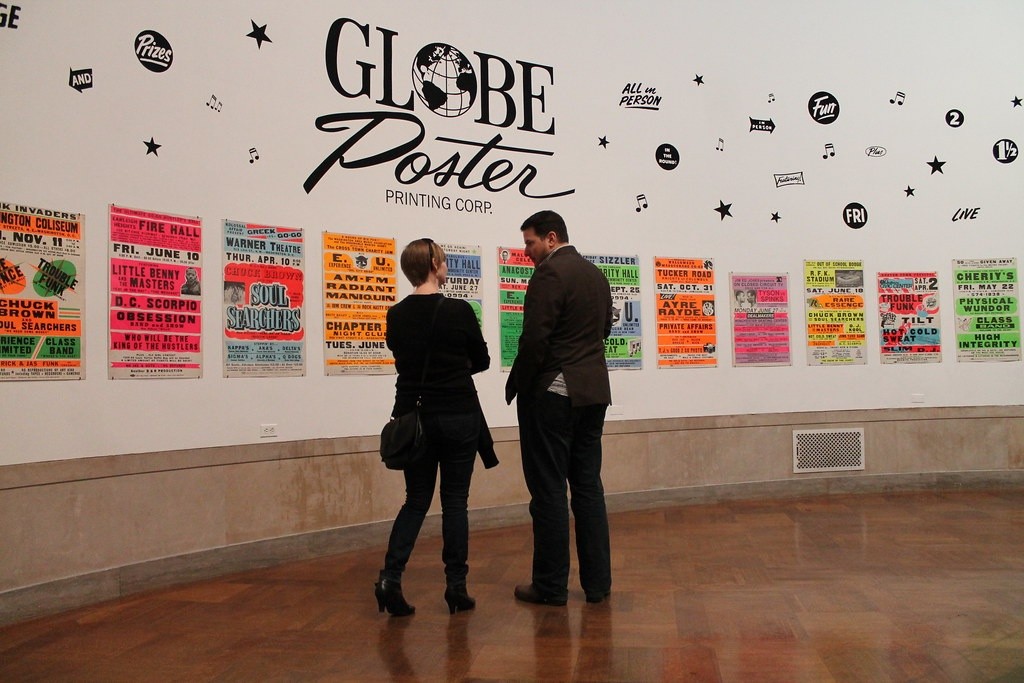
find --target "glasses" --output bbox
[421,238,434,272]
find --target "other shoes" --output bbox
[514,584,567,606]
[586,588,610,603]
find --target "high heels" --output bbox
[444,575,475,614]
[374,569,415,615]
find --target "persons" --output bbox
[224,281,245,305]
[746,290,757,309]
[181,267,201,294]
[734,290,745,309]
[503,210,616,606]
[375,239,499,617]
[501,251,509,261]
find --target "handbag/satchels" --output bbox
[379,396,431,470]
